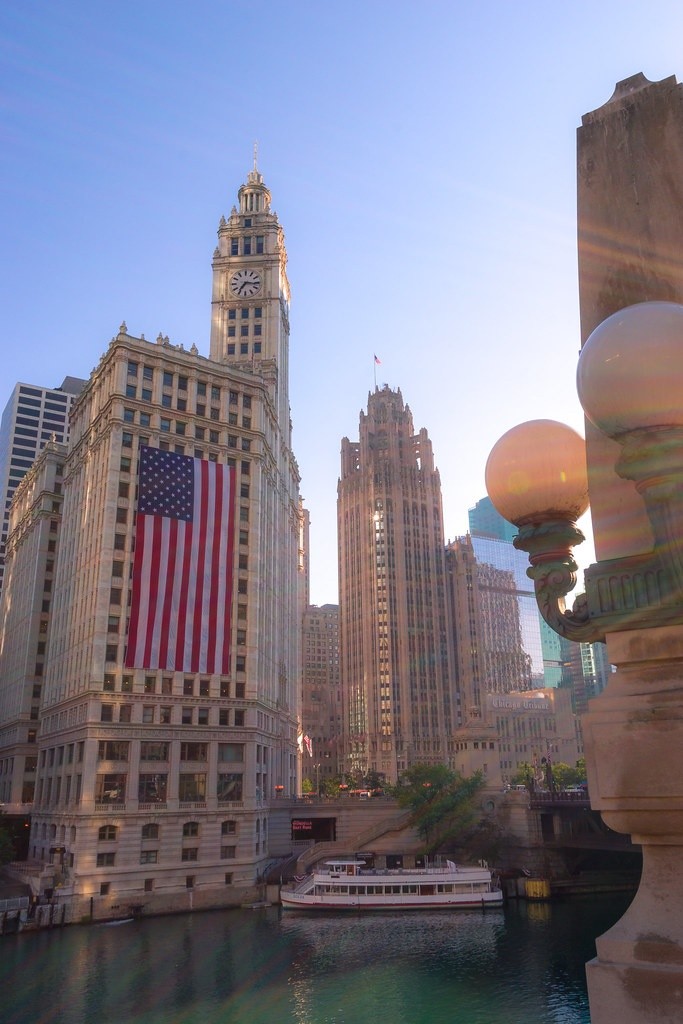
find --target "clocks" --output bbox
[228,268,264,300]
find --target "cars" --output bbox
[359,792,371,798]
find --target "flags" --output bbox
[123,444,238,676]
[304,734,313,758]
[297,733,304,754]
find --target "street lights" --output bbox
[313,745,329,798]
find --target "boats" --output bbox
[280,854,505,910]
